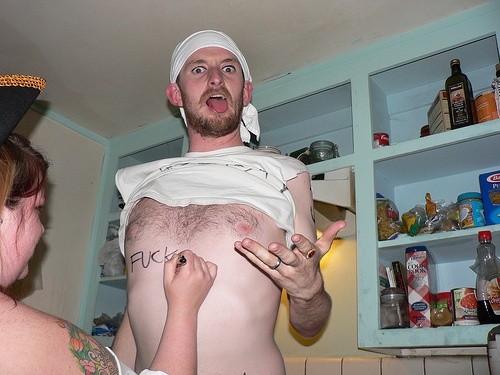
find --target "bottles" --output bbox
[494,64,500,98]
[310,140,335,164]
[457,191,485,229]
[445,59,478,131]
[379,287,409,330]
[476,230,500,325]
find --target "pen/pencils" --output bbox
[177,255,185,265]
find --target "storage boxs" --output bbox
[479,170,500,225]
[405,245,437,328]
[428,90,451,135]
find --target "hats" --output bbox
[0,73,47,145]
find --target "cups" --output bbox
[253,146,282,154]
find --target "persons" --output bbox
[0,132,218,375]
[109,30,347,375]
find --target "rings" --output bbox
[272,259,281,269]
[305,249,315,258]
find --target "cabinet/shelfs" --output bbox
[77,0,500,357]
